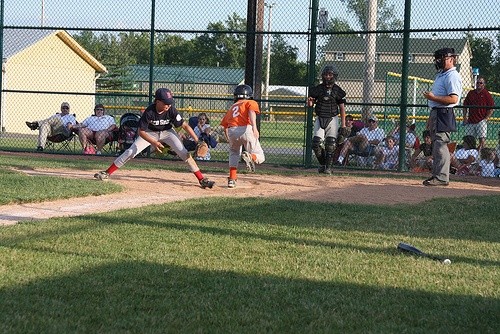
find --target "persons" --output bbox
[462,77,495,148]
[449,135,500,178]
[335,115,434,173]
[423,49,462,186]
[178,113,211,151]
[94,88,215,189]
[308,66,350,174]
[78,104,116,155]
[26,102,76,151]
[222,85,265,188]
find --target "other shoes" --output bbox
[37,146,43,151]
[324,169,333,175]
[334,160,343,166]
[26,121,38,130]
[318,166,325,172]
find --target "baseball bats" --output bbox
[398,243,451,265]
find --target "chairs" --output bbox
[90,114,115,154]
[181,117,210,151]
[46,113,79,154]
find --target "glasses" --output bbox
[476,81,484,84]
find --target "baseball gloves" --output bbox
[195,141,208,158]
[338,126,351,143]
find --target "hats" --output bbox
[441,48,456,58]
[156,88,176,104]
[369,115,378,122]
[61,102,69,108]
[95,104,104,110]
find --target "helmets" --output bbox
[322,66,338,85]
[233,84,253,99]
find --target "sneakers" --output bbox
[241,151,255,173]
[423,176,449,187]
[94,171,109,179]
[199,178,215,189]
[228,179,237,187]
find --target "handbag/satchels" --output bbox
[437,107,457,133]
